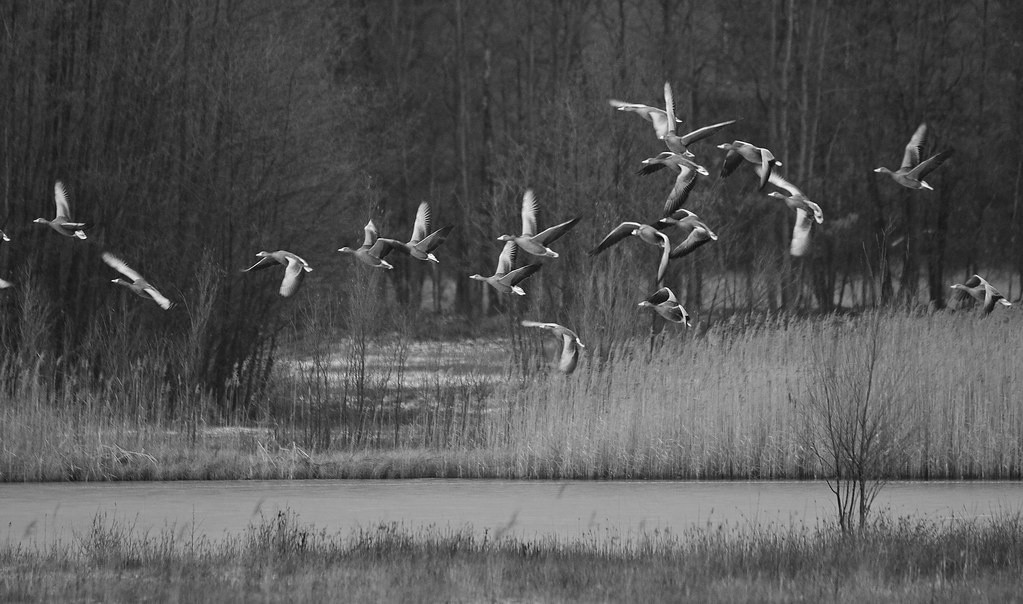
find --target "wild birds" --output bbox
[637,288,694,328]
[606,95,682,141]
[396,197,455,264]
[649,205,719,259]
[718,137,786,191]
[588,219,672,284]
[240,248,317,297]
[338,217,397,272]
[753,166,827,257]
[873,122,955,192]
[947,272,1011,319]
[496,186,583,258]
[31,176,88,243]
[659,79,737,156]
[634,148,710,218]
[521,318,585,376]
[468,237,542,298]
[100,251,180,313]
